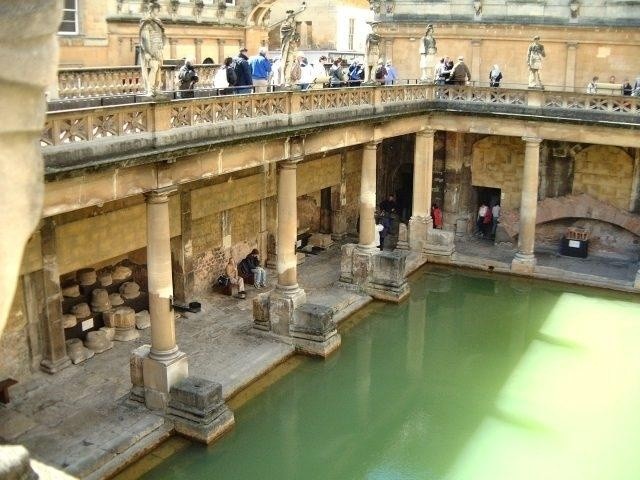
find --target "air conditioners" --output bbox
[552,147,568,158]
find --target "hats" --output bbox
[239,47,247,53]
[251,249,259,255]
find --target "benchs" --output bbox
[0,378,18,404]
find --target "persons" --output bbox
[224,257,245,295]
[436,55,472,88]
[375,219,384,248]
[177,43,401,95]
[379,215,389,251]
[139,2,166,97]
[366,24,383,81]
[632,78,640,109]
[380,194,397,213]
[587,76,601,94]
[488,63,504,99]
[421,21,438,81]
[609,76,616,84]
[491,201,502,235]
[243,247,267,291]
[280,8,303,86]
[526,34,545,90]
[623,77,632,108]
[431,202,444,229]
[474,201,490,237]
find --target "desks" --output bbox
[560,237,588,258]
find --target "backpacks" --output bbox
[238,259,251,277]
[231,58,244,85]
[174,74,183,87]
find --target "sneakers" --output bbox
[253,282,261,290]
[260,281,267,287]
[238,291,247,299]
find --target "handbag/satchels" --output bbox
[218,275,228,286]
[350,70,362,80]
[331,65,344,80]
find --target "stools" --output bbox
[240,259,253,285]
[223,283,239,297]
